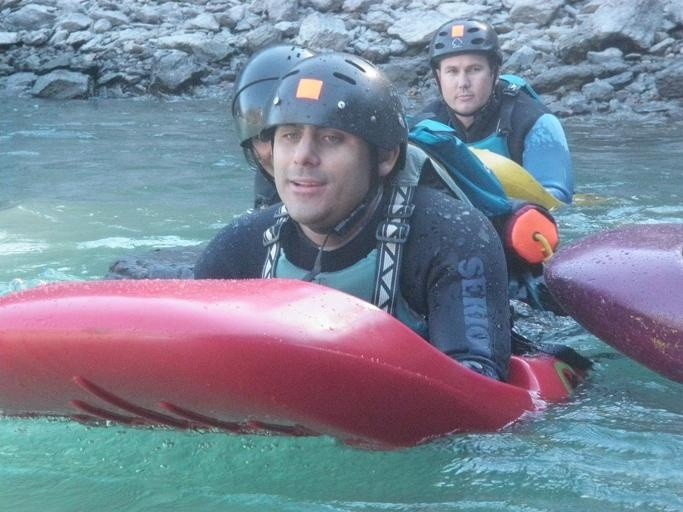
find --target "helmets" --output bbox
[428,18,501,64]
[229,42,408,171]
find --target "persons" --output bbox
[232,43,317,211]
[407,19,575,204]
[194,53,513,383]
